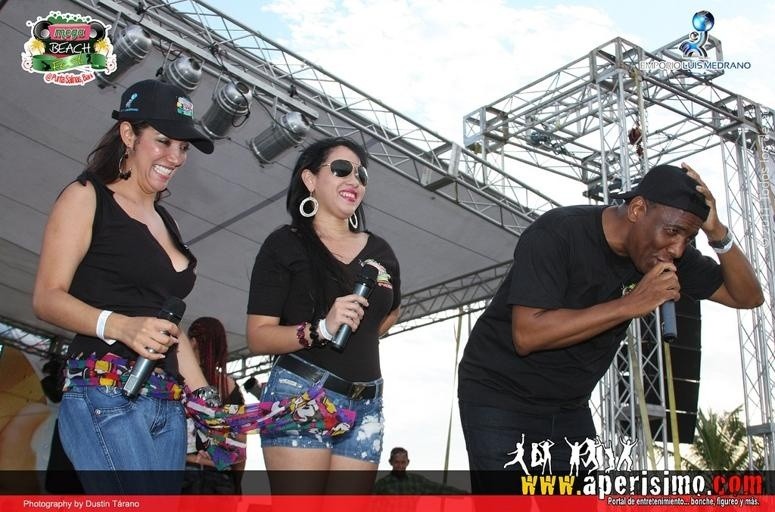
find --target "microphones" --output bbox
[660,268,679,344]
[121,295,187,399]
[331,264,379,352]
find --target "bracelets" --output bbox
[309,311,329,349]
[714,240,737,255]
[297,320,309,350]
[709,228,733,248]
[96,310,117,346]
[191,386,222,409]
[318,319,332,342]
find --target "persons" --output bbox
[458,162,766,494]
[245,137,400,495]
[376,448,468,495]
[31,79,223,494]
[188,317,246,495]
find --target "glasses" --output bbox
[322,159,368,186]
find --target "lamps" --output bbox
[86,15,323,164]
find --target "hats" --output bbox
[112,79,214,154]
[611,164,710,223]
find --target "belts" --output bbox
[275,354,382,404]
